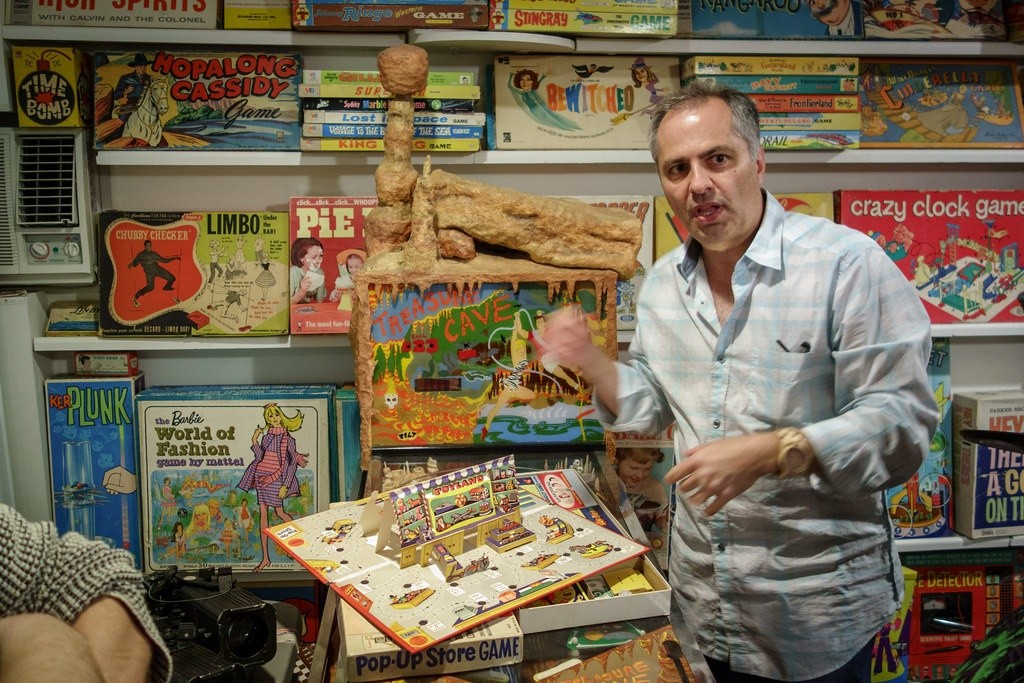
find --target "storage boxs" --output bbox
[91,50,301,152]
[39,189,1024,683]
[12,46,89,129]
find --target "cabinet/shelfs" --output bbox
[0,24,1024,552]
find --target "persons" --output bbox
[540,86,942,683]
[290,237,367,305]
[128,238,182,307]
[614,446,668,533]
[0,502,173,683]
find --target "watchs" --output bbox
[775,427,812,480]
